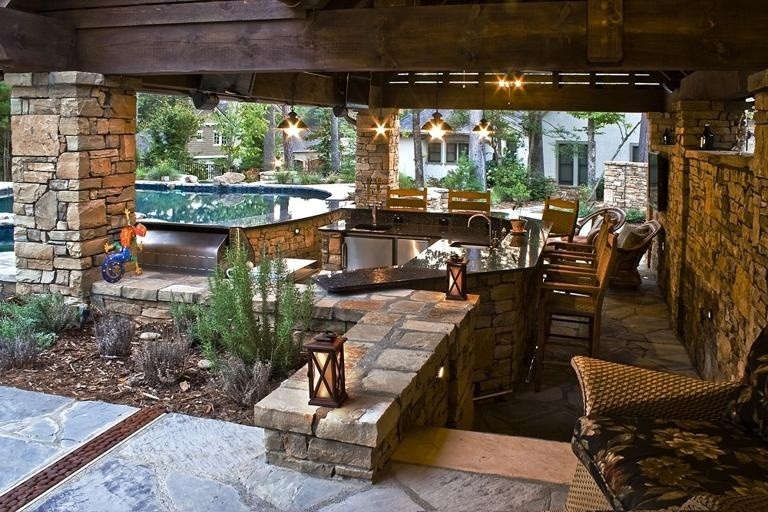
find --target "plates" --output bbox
[510,229,528,234]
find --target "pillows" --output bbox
[617,221,651,248]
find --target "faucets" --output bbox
[468,213,492,246]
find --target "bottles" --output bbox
[699,122,715,151]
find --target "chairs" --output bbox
[534,196,626,392]
[385,185,492,211]
[607,220,663,287]
[562,322,767,512]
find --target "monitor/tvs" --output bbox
[647,152,663,211]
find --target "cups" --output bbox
[511,219,528,231]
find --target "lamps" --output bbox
[274,75,312,136]
[302,329,349,407]
[444,253,471,301]
[419,74,499,137]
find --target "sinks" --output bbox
[450,240,490,249]
